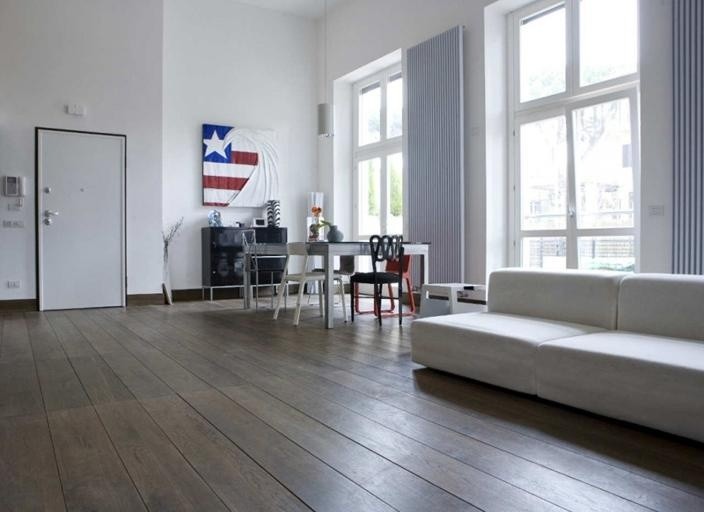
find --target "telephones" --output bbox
[3,176,26,197]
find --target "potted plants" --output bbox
[320,220,344,242]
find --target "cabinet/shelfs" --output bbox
[201,226,288,303]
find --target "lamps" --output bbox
[318,0,334,137]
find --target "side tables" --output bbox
[419,282,486,319]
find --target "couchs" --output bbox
[410,267,703,442]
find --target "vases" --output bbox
[309,234,320,242]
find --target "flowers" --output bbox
[309,206,321,233]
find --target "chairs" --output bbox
[350,234,405,326]
[273,240,348,328]
[308,253,355,304]
[355,255,416,317]
[240,229,288,314]
